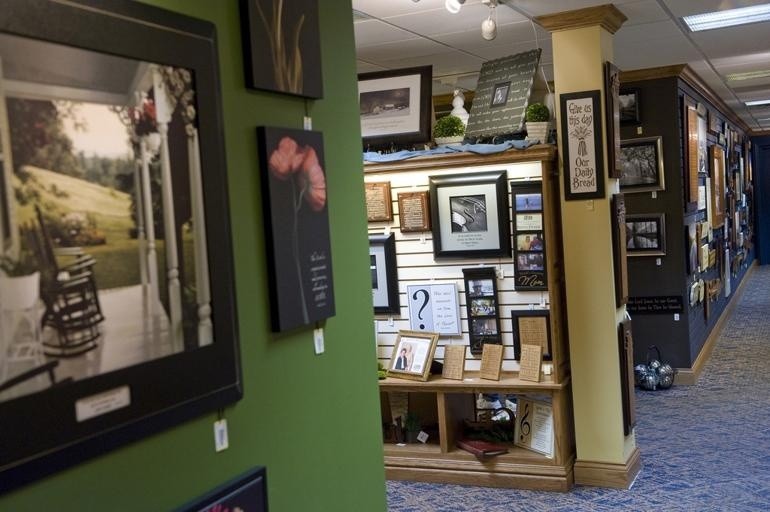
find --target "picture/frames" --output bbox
[357,64,433,143]
[559,89,606,201]
[602,58,671,308]
[362,169,543,380]
[0,2,249,497]
[513,395,555,461]
[617,318,638,437]
[680,97,754,326]
[165,465,271,512]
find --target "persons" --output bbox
[469,281,493,315]
[520,234,543,266]
[394,349,408,369]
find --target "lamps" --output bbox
[445,0,499,41]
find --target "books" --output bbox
[456,437,511,458]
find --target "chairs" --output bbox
[0,202,105,386]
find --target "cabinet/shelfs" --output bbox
[379,384,573,492]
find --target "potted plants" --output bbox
[434,114,468,146]
[524,103,552,141]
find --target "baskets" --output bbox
[634,345,679,392]
